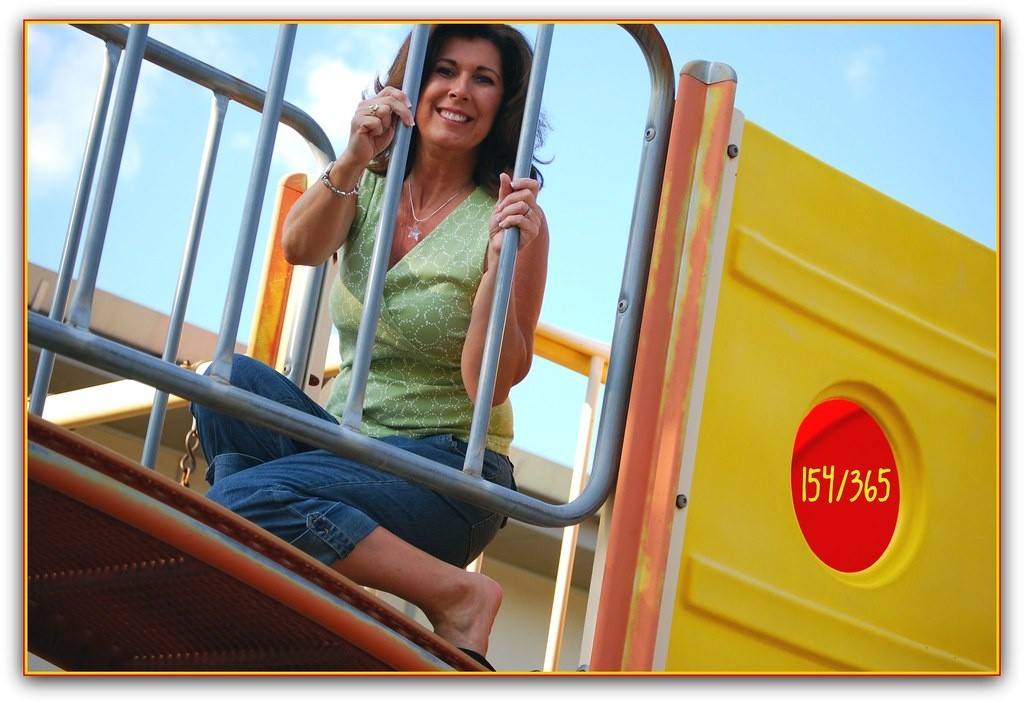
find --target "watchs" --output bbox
[321,160,360,197]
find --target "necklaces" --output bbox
[407,178,466,242]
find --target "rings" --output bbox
[368,104,379,117]
[524,208,534,217]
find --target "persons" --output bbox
[187,22,550,671]
